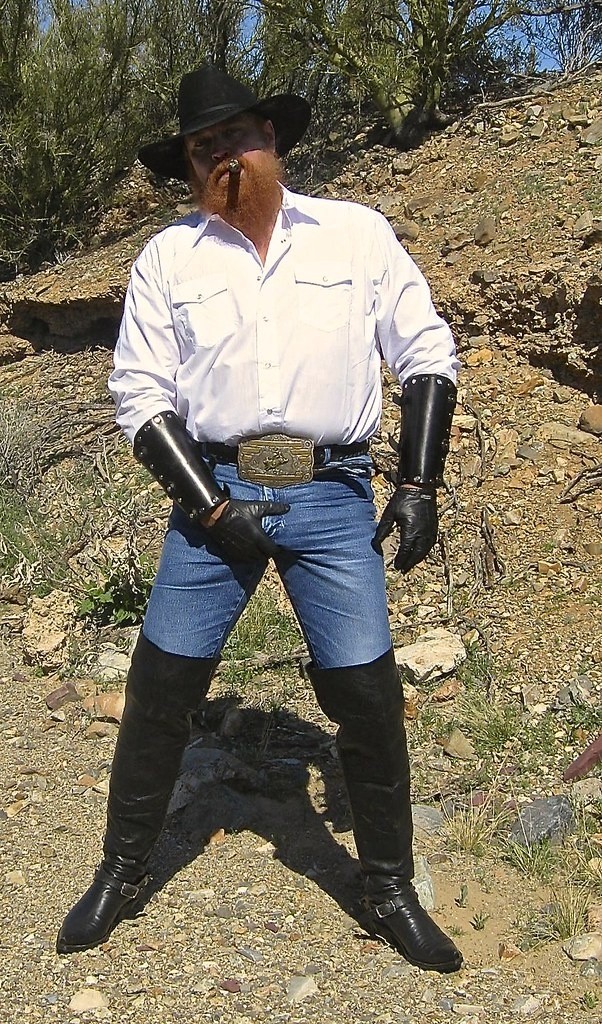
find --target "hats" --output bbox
[138,67,311,183]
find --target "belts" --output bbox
[199,434,369,489]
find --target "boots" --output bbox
[305,648,461,970]
[56,630,221,952]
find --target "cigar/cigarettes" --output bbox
[227,159,242,173]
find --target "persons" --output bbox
[53,59,472,978]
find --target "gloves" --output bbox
[372,487,439,574]
[205,498,291,563]
